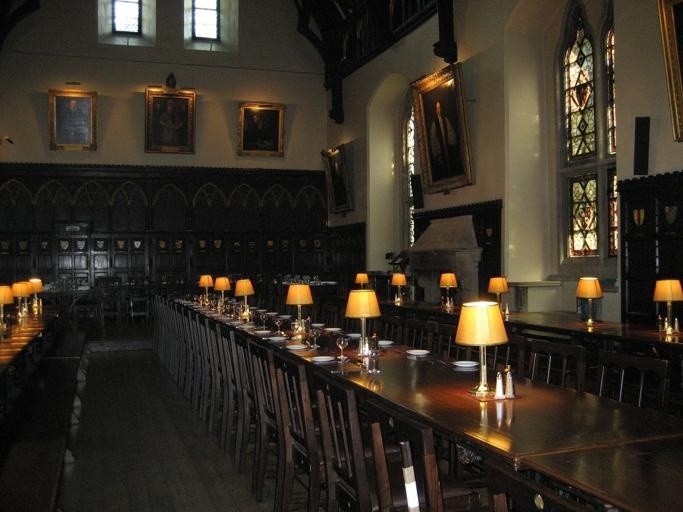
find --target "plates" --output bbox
[269,337,286,341]
[286,345,308,350]
[324,328,341,331]
[347,333,362,338]
[311,356,335,362]
[406,349,430,355]
[254,330,272,334]
[451,360,480,367]
[312,323,325,327]
[378,340,393,346]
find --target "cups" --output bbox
[260,314,267,330]
[276,318,282,333]
[337,338,348,359]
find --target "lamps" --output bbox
[213,277,230,302]
[200,274,213,298]
[392,273,406,297]
[488,277,509,304]
[345,289,380,345]
[29,278,45,300]
[0,285,14,328]
[13,281,33,313]
[234,278,255,305]
[439,273,457,304]
[576,277,603,325]
[165,72,175,90]
[653,279,683,327]
[286,284,313,321]
[456,301,509,392]
[355,273,370,290]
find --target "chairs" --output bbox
[437,323,472,363]
[311,372,474,512]
[234,331,334,474]
[485,455,592,512]
[488,333,527,378]
[128,275,149,320]
[95,277,123,327]
[597,348,670,412]
[273,351,404,512]
[403,318,439,354]
[365,398,489,511]
[342,308,360,332]
[66,277,98,326]
[527,339,587,394]
[373,313,404,344]
[150,292,312,441]
[249,340,361,503]
[322,304,338,328]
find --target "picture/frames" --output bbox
[655,0,681,145]
[410,63,475,194]
[47,87,98,151]
[321,144,355,214]
[236,101,285,158]
[144,88,196,154]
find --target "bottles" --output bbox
[504,364,516,395]
[505,303,510,314]
[656,315,663,332]
[494,371,505,399]
[504,371,515,398]
[450,298,453,306]
[664,317,668,330]
[372,333,379,350]
[674,317,679,332]
